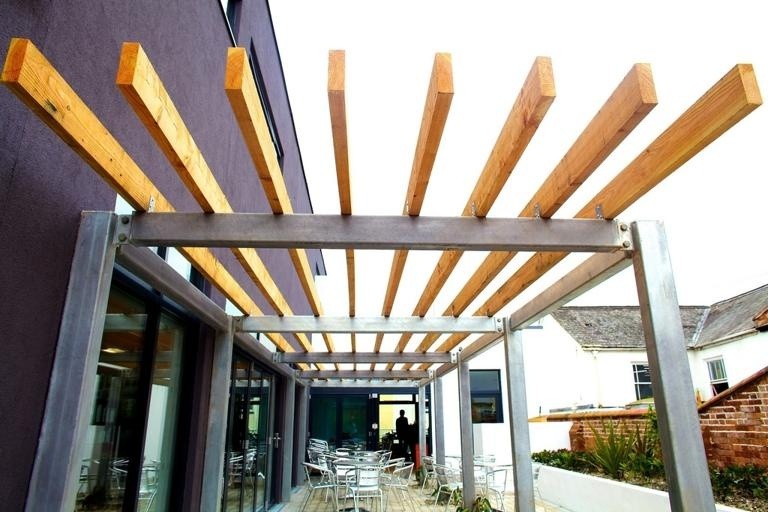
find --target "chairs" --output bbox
[301,438,546,512]
[77,456,161,512]
[229,445,256,497]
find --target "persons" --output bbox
[396,409,409,450]
